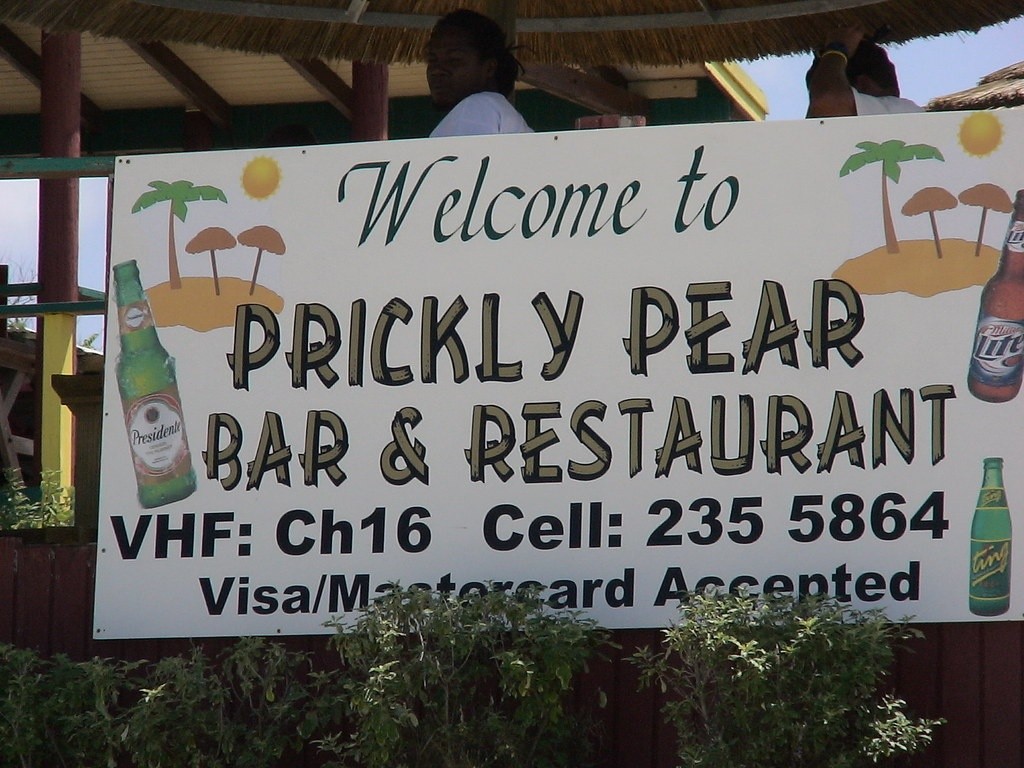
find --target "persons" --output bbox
[805,24,923,119]
[425,10,535,138]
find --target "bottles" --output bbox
[968,456,1013,617]
[966,187,1024,404]
[112,258,198,509]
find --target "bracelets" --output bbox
[822,43,849,56]
[821,50,847,62]
